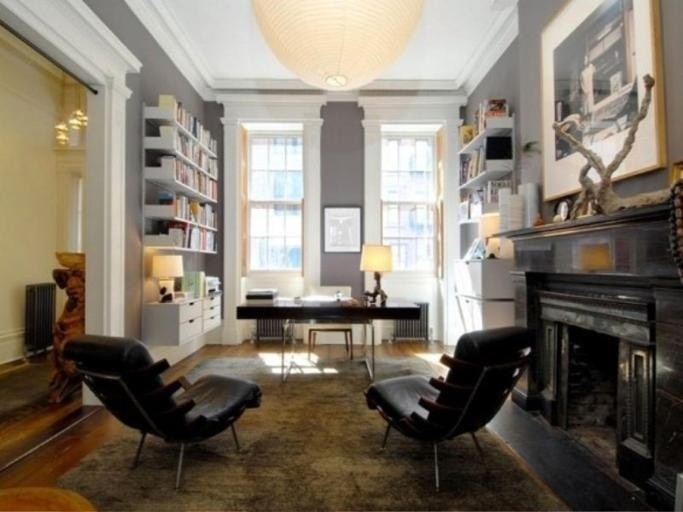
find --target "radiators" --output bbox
[392,302,429,342]
[255,317,289,341]
[24,283,56,351]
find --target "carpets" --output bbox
[54,356,572,510]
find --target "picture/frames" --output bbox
[538,0,670,206]
[321,204,363,255]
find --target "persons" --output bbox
[576,54,598,126]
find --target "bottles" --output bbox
[336,291,341,301]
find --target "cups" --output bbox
[488,180,505,202]
[497,182,539,233]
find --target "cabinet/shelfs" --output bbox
[138,101,219,256]
[456,125,517,226]
[451,257,514,345]
[142,292,222,349]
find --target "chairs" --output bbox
[308,285,355,361]
[362,325,537,491]
[59,333,262,491]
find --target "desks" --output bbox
[236,297,422,383]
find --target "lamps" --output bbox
[360,244,393,305]
[249,0,424,92]
[151,253,185,302]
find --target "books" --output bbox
[456,97,537,263]
[181,271,206,300]
[154,95,217,251]
[245,288,278,306]
